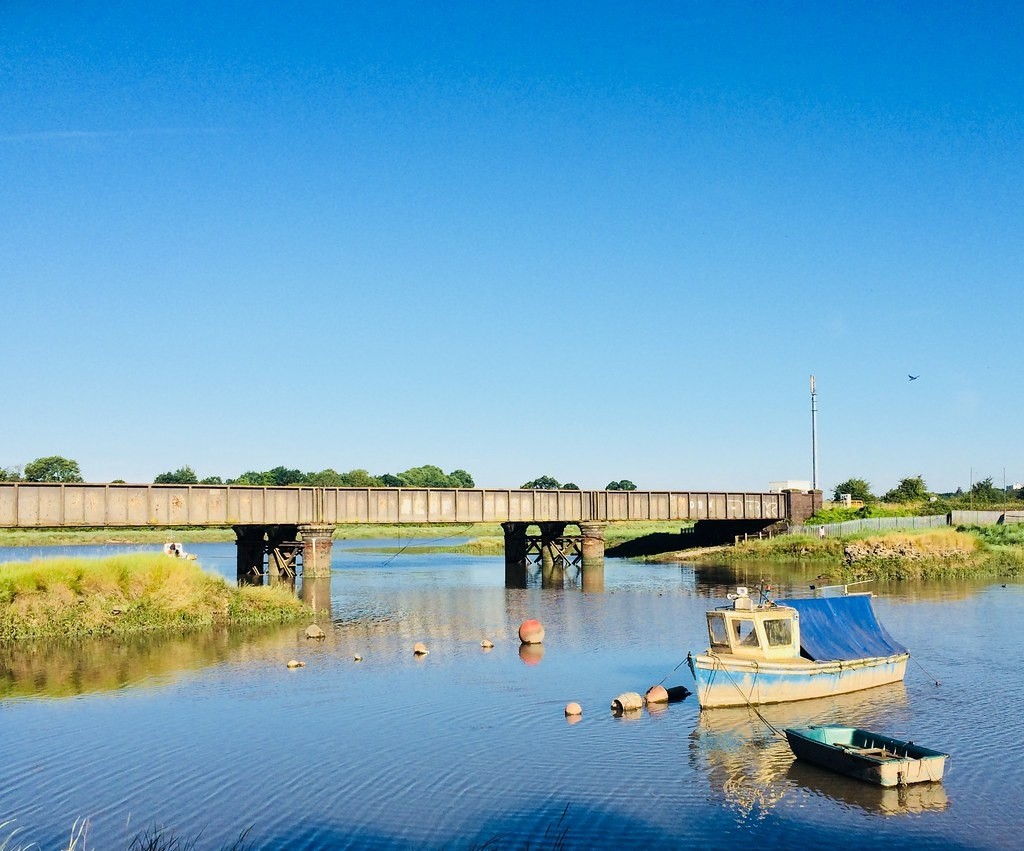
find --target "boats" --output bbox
[781,724,951,788]
[687,579,910,709]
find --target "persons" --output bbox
[170,542,175,550]
[820,526,825,539]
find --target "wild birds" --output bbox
[908,374,920,382]
[1002,584,1006,588]
